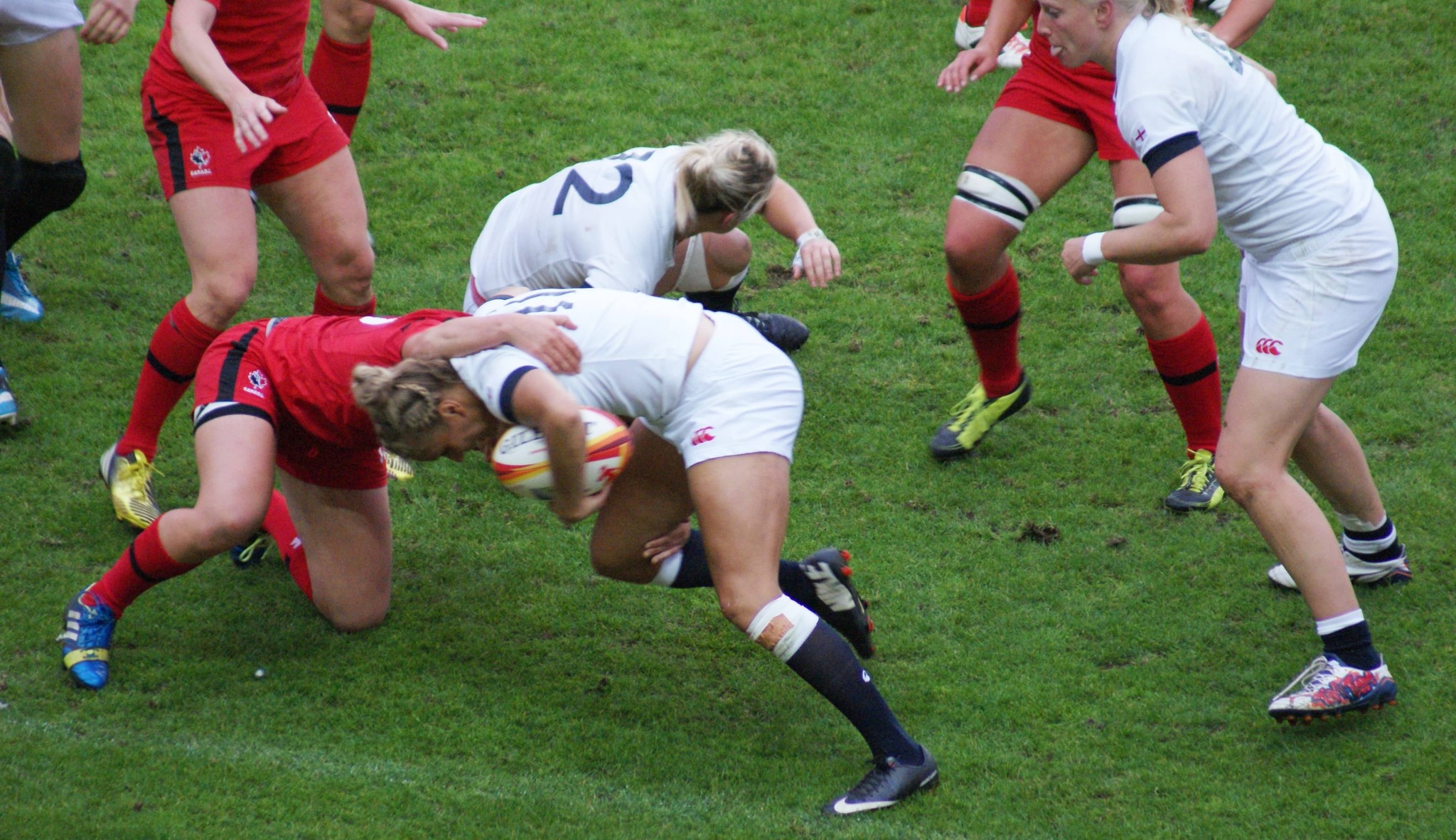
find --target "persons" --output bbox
[1030,0,1414,731]
[462,124,846,358]
[352,285,943,823]
[0,0,144,429]
[304,0,378,147]
[924,0,1276,518]
[953,0,1033,73]
[61,300,584,689]
[99,0,489,543]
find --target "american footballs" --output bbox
[490,407,633,500]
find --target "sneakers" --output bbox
[58,580,117,690]
[379,446,414,481]
[1163,448,1227,511]
[796,547,876,659]
[733,309,809,353]
[928,370,1034,456]
[97,436,162,531]
[0,249,44,323]
[0,362,17,428]
[954,5,1031,66]
[822,743,939,817]
[1267,651,1396,727]
[1267,544,1414,596]
[231,521,274,569]
[1192,0,1231,17]
